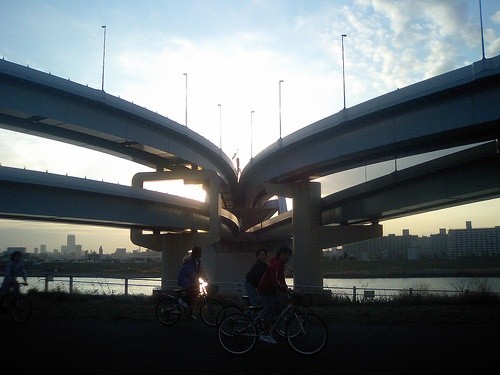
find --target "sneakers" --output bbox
[260,335,278,343]
[280,329,285,334]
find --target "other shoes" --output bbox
[178,297,188,308]
[191,315,203,321]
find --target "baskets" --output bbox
[206,285,219,297]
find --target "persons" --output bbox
[177,247,208,320]
[244,248,268,318]
[1,251,28,307]
[258,246,293,344]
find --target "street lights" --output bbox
[249,110,255,159]
[278,79,285,139]
[182,73,188,129]
[218,103,222,151]
[341,34,348,108]
[102,25,107,91]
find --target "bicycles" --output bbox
[0,282,33,323]
[216,289,329,357]
[154,279,226,327]
